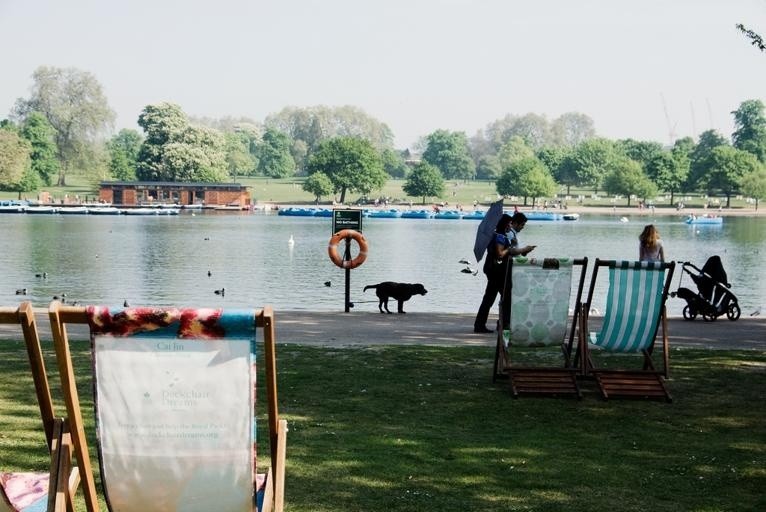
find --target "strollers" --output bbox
[677,255,741,322]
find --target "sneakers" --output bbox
[474,325,493,333]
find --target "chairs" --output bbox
[494,257,676,401]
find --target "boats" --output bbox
[686,215,723,224]
[0,199,279,215]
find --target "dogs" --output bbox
[363,281,428,314]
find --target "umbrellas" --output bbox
[472,198,504,262]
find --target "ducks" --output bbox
[214,287,226,294]
[323,280,331,287]
[16,288,27,296]
[461,266,475,273]
[458,257,471,264]
[35,271,48,278]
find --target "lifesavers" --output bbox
[329,229,368,268]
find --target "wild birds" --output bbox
[123,299,129,307]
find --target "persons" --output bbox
[430,201,463,215]
[374,194,390,207]
[514,204,518,213]
[544,200,548,212]
[507,212,534,257]
[639,224,666,262]
[473,200,478,209]
[639,200,644,213]
[474,214,519,334]
